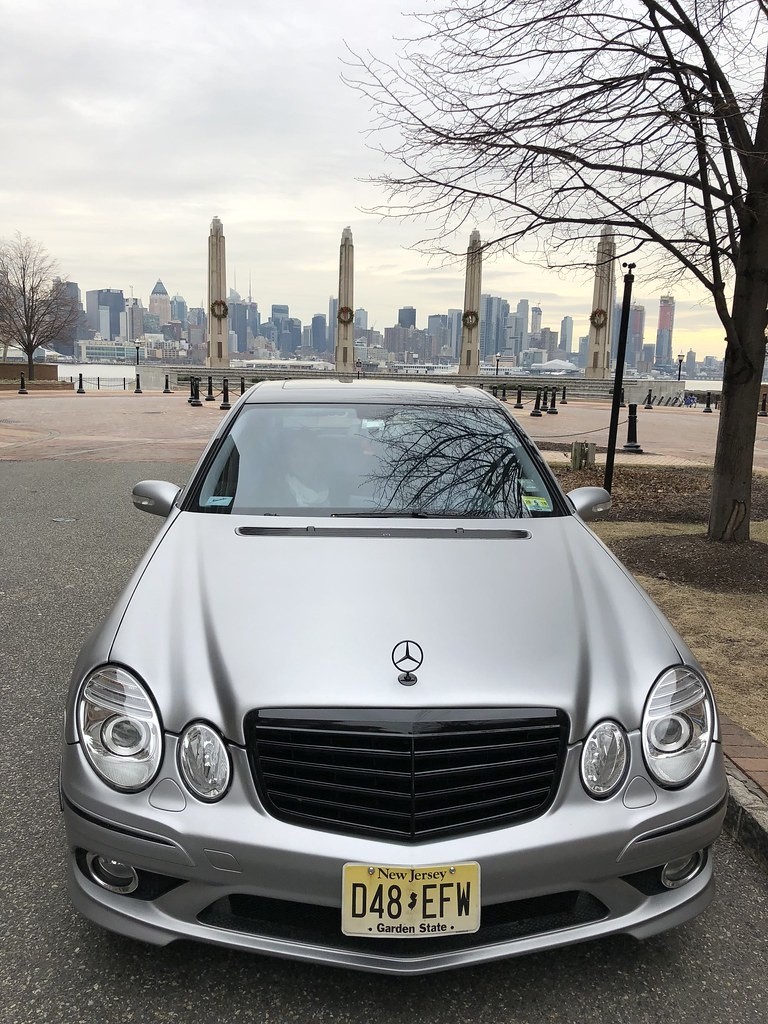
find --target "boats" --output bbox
[394,363,513,375]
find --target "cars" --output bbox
[60,377,728,976]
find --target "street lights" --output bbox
[134,338,141,365]
[678,351,685,380]
[496,353,501,374]
